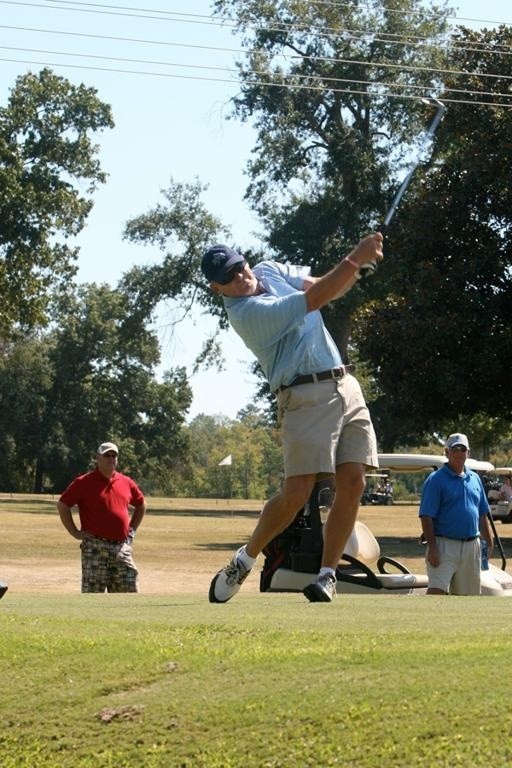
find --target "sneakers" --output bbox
[304,572,336,602]
[209,546,252,602]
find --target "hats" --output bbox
[97,442,119,455]
[201,244,244,282]
[446,433,469,450]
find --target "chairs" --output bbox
[323,515,429,594]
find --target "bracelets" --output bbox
[344,256,359,270]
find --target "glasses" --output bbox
[215,260,246,284]
[449,447,467,451]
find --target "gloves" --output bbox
[127,526,135,545]
[353,257,379,280]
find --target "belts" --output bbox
[274,364,356,393]
[434,536,478,541]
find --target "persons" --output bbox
[58,443,145,592]
[422,432,493,596]
[500,478,511,502]
[201,231,386,603]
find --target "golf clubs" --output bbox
[358,99,445,280]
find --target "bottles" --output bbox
[480,540,489,570]
